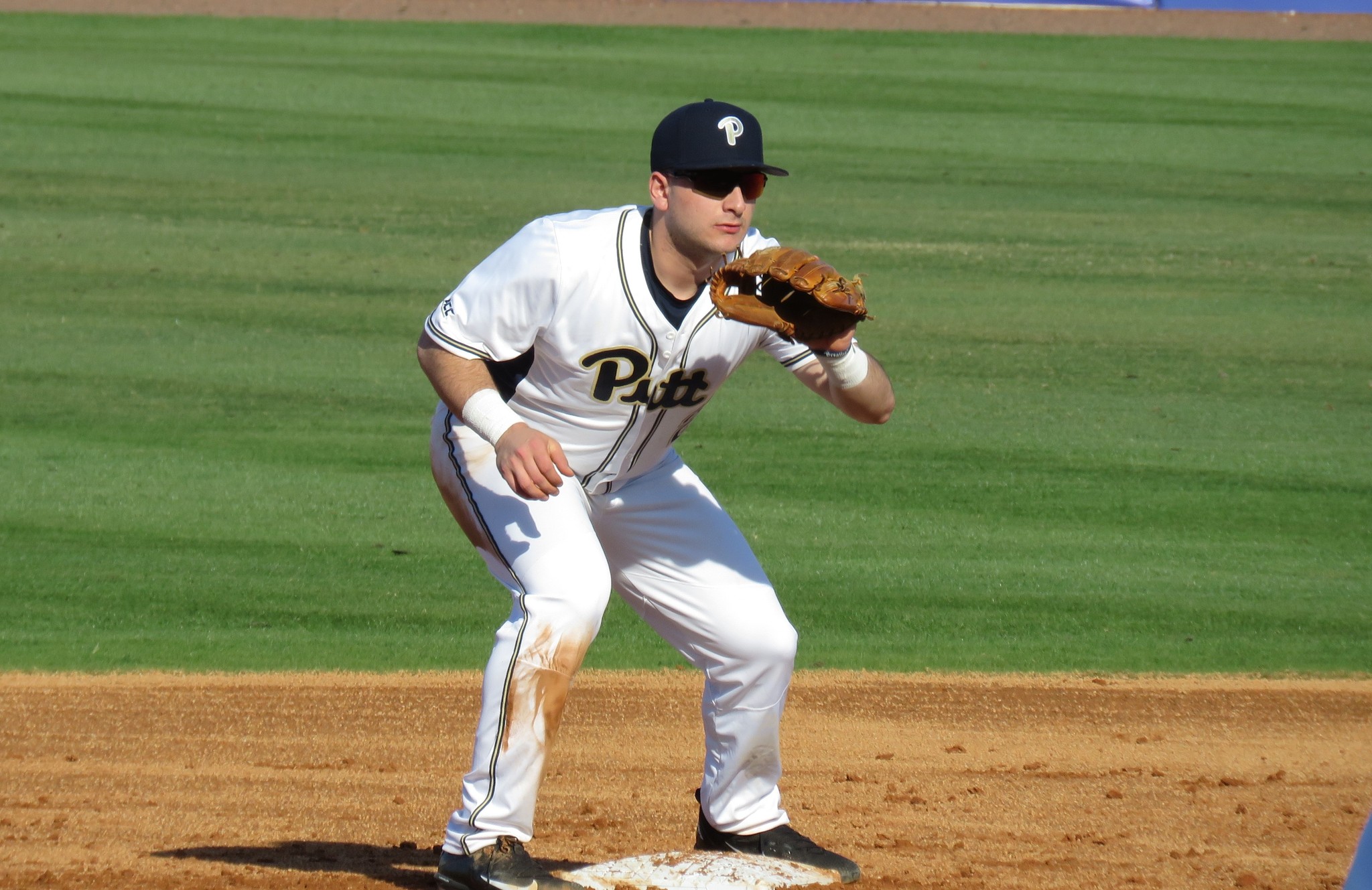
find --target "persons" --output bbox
[417,99,896,890]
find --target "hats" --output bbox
[649,96,791,177]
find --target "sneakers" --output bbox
[434,836,582,887]
[693,788,861,883]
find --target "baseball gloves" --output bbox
[709,246,868,346]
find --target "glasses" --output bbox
[687,172,768,204]
